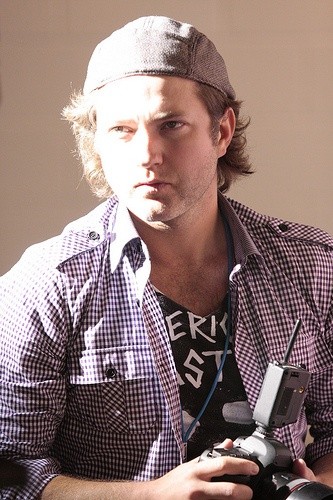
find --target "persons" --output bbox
[0,15,333,500]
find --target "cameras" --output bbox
[198,318,333,500]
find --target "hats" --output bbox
[83,15,237,102]
